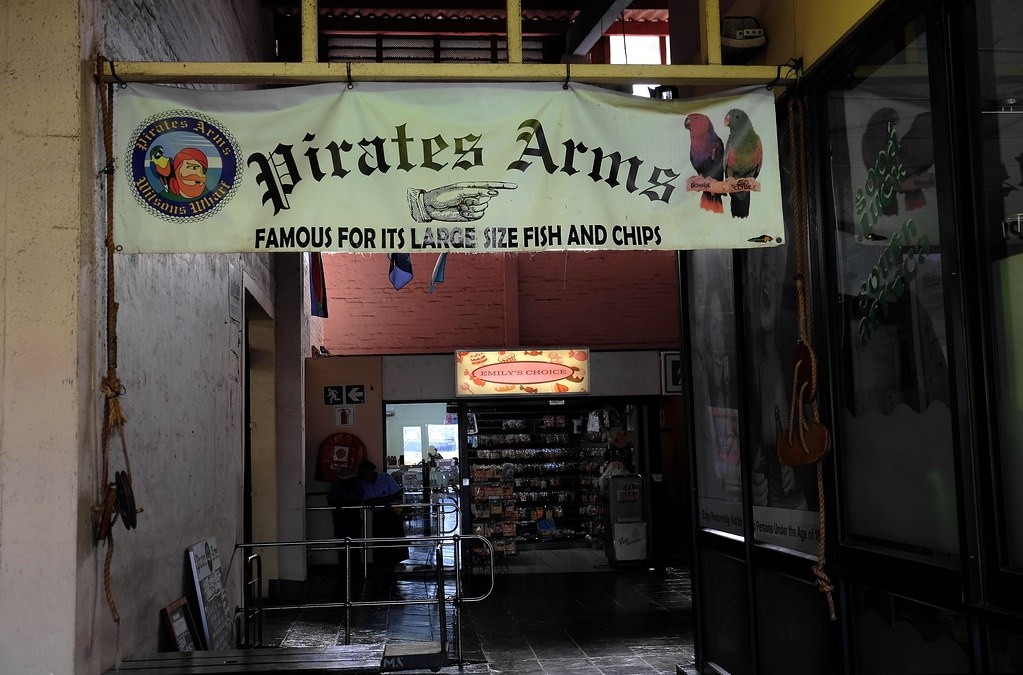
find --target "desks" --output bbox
[103,641,386,675]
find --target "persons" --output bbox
[358,461,405,587]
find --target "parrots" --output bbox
[724,109,763,219]
[685,113,727,213]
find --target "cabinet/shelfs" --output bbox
[387,459,431,536]
[469,466,519,567]
